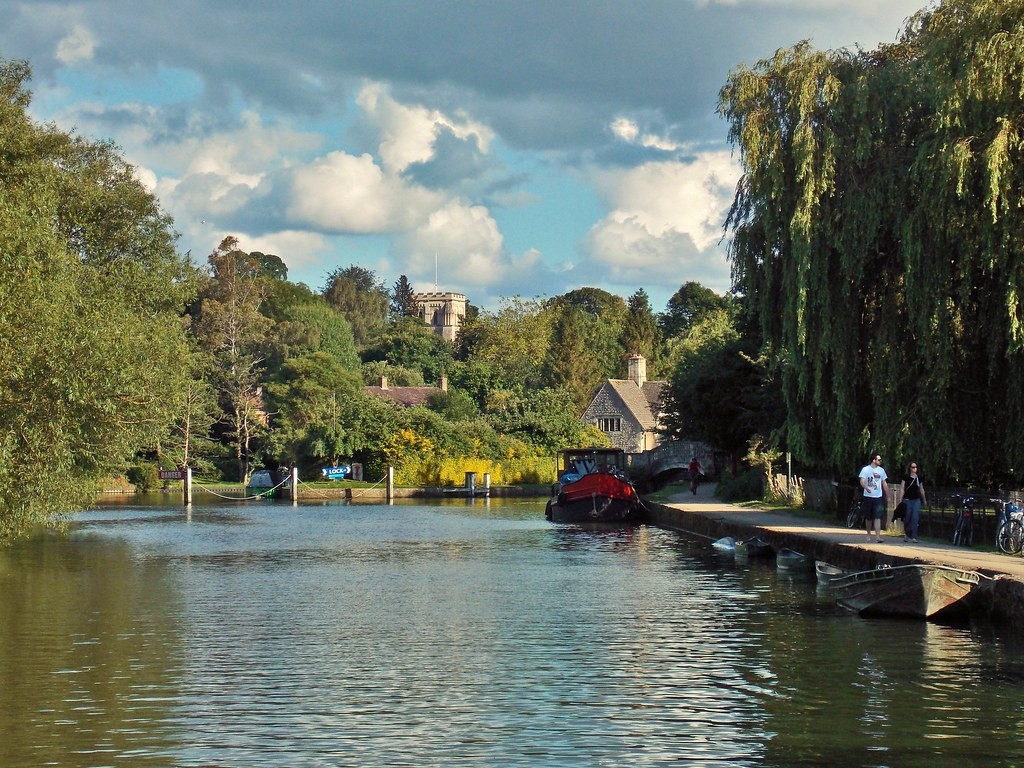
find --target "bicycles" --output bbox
[845,486,864,529]
[953,494,978,547]
[688,472,704,496]
[990,497,1024,554]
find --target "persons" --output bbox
[688,456,701,490]
[858,453,891,543]
[899,460,928,543]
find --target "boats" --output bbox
[545,445,643,523]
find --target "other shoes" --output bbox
[876,538,884,542]
[904,536,908,542]
[912,539,918,543]
[867,537,871,543]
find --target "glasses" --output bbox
[909,466,918,468]
[875,459,882,461]
